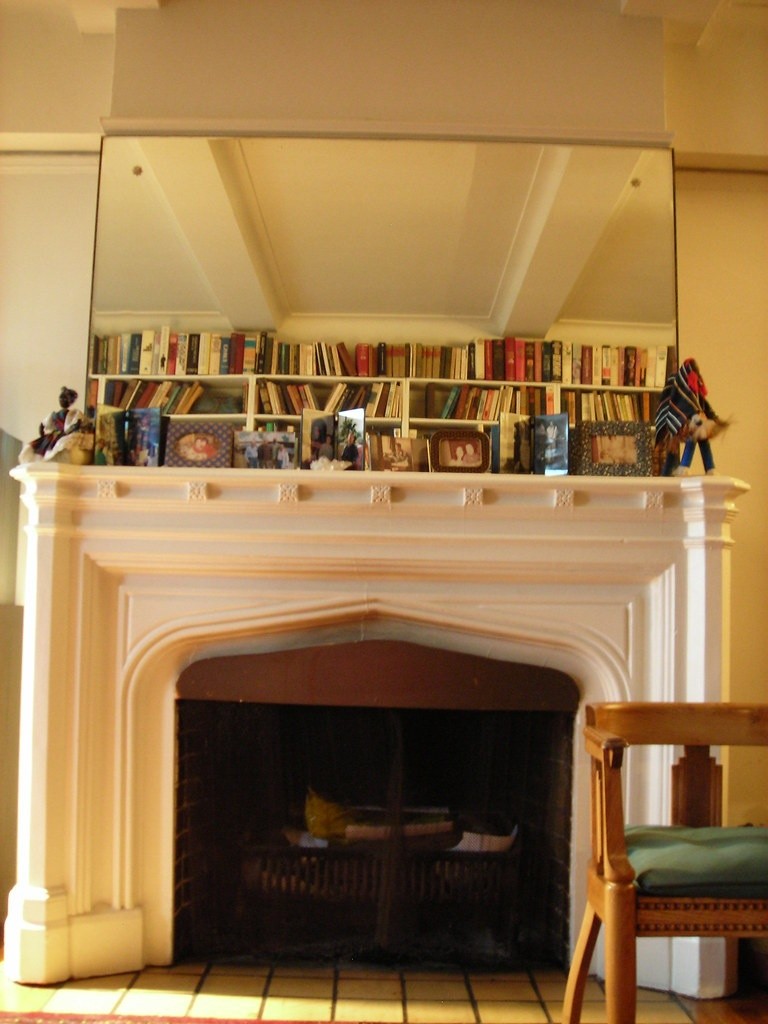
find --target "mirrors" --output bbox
[82,130,684,443]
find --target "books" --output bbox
[92,327,676,388]
[258,423,298,468]
[394,428,417,438]
[87,377,641,427]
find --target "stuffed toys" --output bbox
[655,358,731,476]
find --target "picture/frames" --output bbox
[164,421,234,467]
[429,427,490,473]
[575,419,658,477]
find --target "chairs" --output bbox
[558,698,768,1024]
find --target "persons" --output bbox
[94,412,152,467]
[600,435,635,464]
[245,437,289,469]
[187,433,216,460]
[310,426,334,462]
[514,421,530,472]
[538,420,558,438]
[161,354,166,371]
[30,385,78,457]
[339,433,362,470]
[387,443,409,470]
[449,444,480,465]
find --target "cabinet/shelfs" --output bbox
[88,327,677,437]
